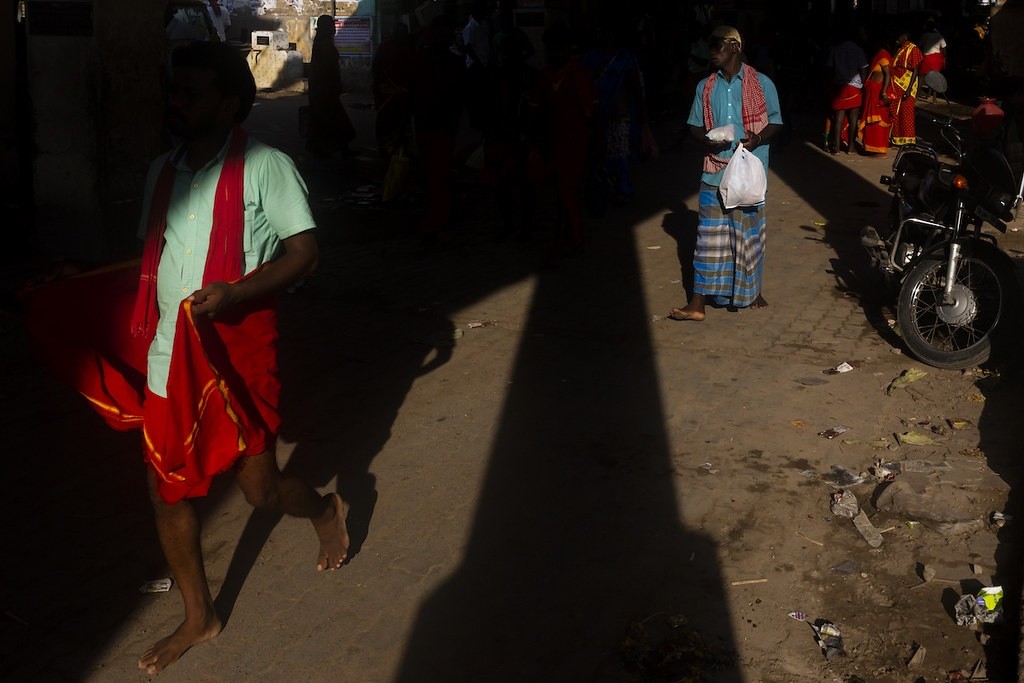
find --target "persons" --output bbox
[749,20,794,151]
[28,43,348,679]
[372,0,708,261]
[304,16,361,159]
[669,26,782,320]
[821,14,947,157]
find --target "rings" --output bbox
[904,95,906,97]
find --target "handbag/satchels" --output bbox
[718,140,768,210]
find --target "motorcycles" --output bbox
[858,70,1024,371]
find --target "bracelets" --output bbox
[880,94,886,97]
[756,135,761,146]
